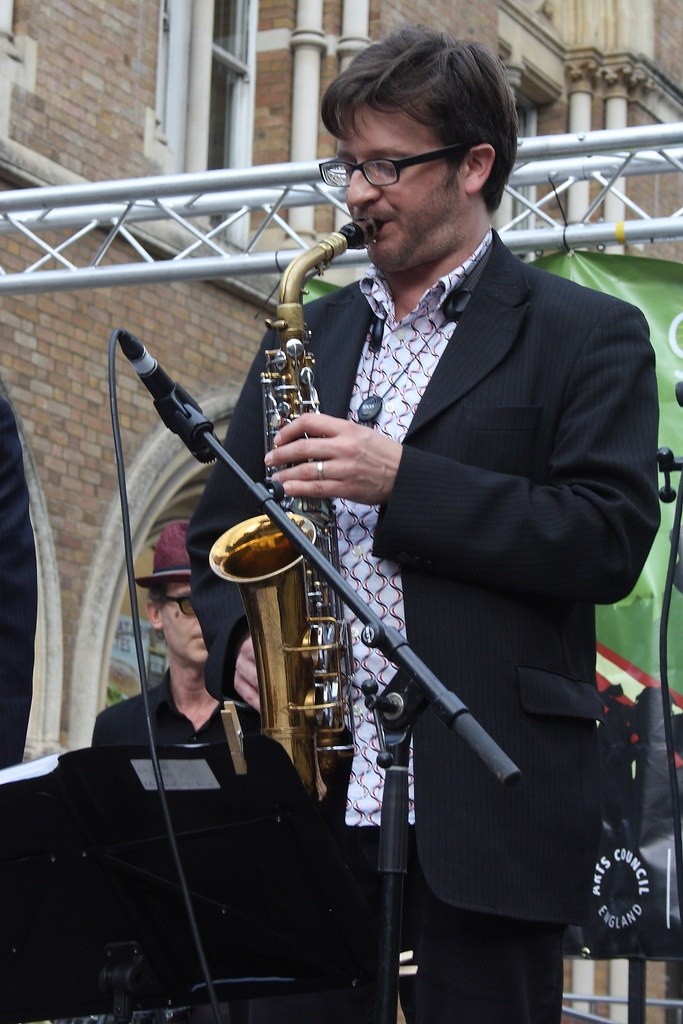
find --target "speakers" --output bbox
[0,736,376,1024]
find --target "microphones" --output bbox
[119,323,223,466]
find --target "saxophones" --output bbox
[208,214,387,805]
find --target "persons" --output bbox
[185,22,661,1023]
[90,522,240,749]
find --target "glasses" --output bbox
[164,595,195,616]
[318,142,474,187]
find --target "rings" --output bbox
[316,462,324,484]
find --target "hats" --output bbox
[134,521,192,588]
